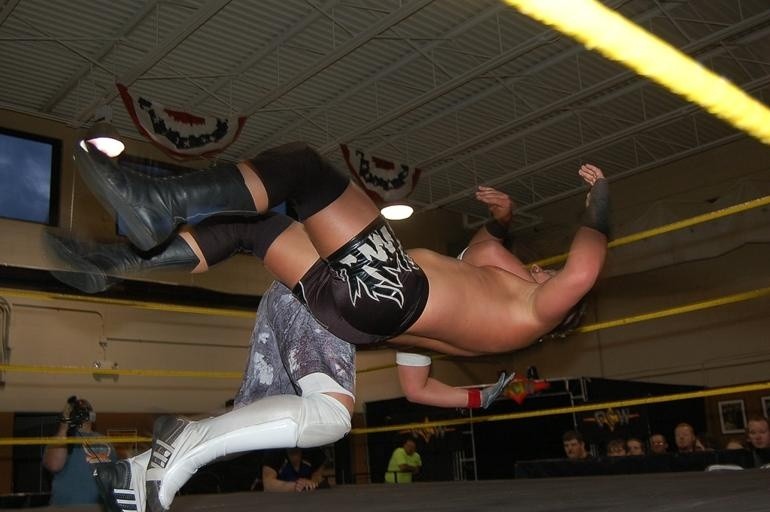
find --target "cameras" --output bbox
[62,394,88,438]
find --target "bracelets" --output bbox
[465,388,481,409]
[485,215,508,239]
[58,415,70,423]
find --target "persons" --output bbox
[607,438,626,456]
[649,434,669,457]
[673,422,697,452]
[312,438,357,486]
[42,122,614,359]
[380,436,423,484]
[91,276,519,512]
[38,394,120,505]
[745,414,770,449]
[261,447,328,492]
[721,435,745,449]
[559,430,595,459]
[625,436,645,456]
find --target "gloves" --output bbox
[575,176,613,240]
[479,370,517,409]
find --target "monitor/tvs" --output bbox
[0,128,64,232]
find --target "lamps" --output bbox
[380,188,415,221]
[79,122,125,158]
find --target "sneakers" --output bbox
[93,458,145,512]
[142,412,198,512]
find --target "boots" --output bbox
[36,228,205,295]
[73,140,258,255]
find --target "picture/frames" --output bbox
[718,399,748,435]
[761,395,770,421]
